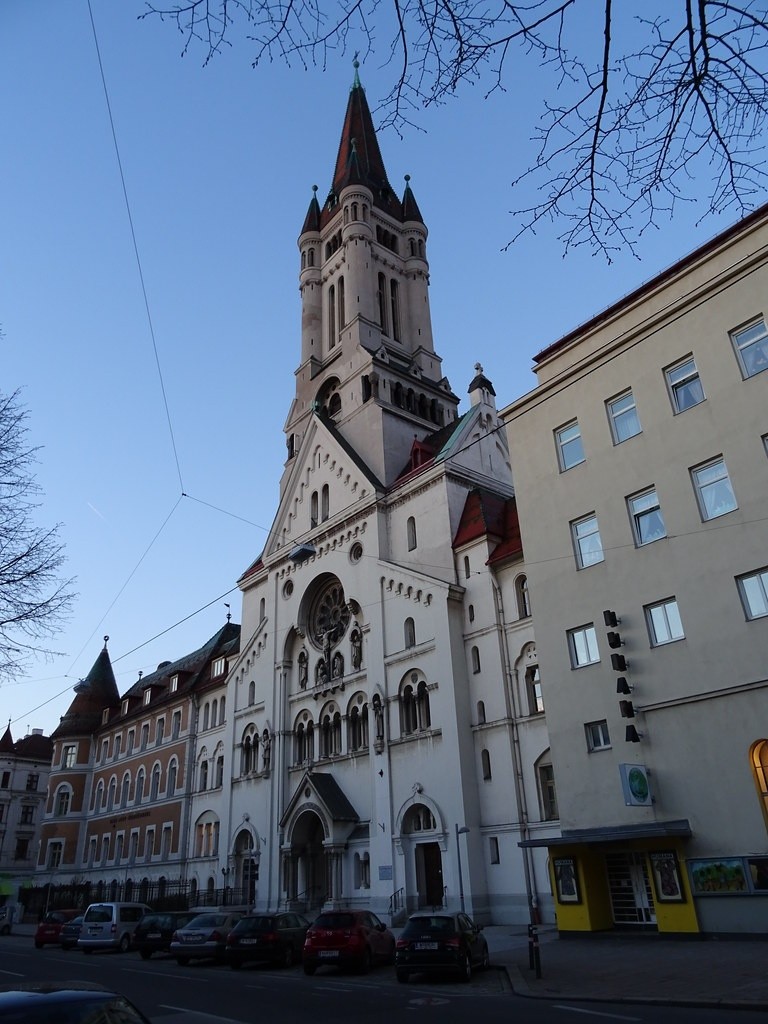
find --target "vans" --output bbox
[78,902,153,952]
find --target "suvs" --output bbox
[303,909,396,975]
[394,911,490,983]
[226,912,311,969]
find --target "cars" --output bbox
[34,911,245,966]
[0,981,153,1024]
